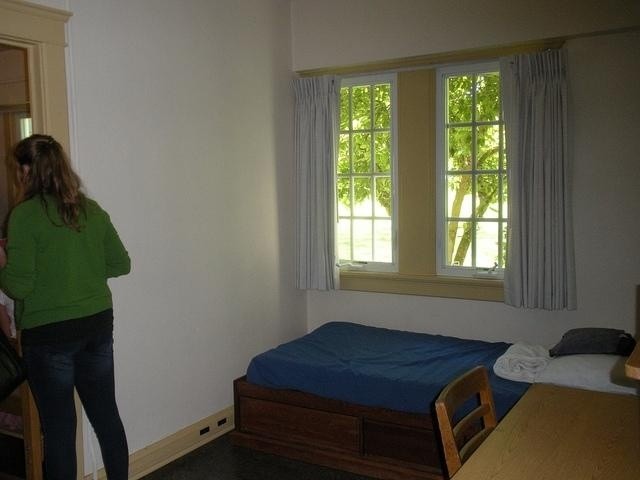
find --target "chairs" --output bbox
[435,364,499,477]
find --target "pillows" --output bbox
[536,327,640,396]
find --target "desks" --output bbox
[449,338,640,480]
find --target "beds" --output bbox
[231,321,531,480]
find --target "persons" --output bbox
[0,132,132,480]
